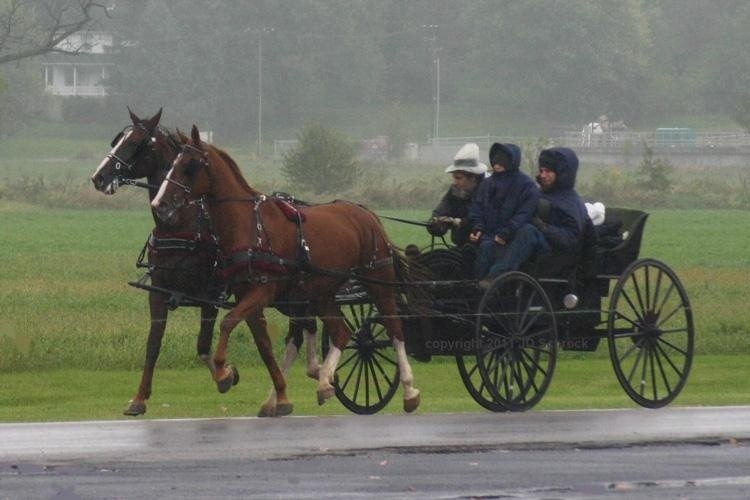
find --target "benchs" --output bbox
[452,208,648,297]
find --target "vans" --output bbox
[636,127,694,148]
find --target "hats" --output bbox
[445,142,488,176]
[538,150,556,172]
[491,148,512,170]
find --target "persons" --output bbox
[458,141,539,261]
[401,139,494,260]
[469,145,596,291]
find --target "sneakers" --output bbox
[475,278,493,294]
[455,243,478,280]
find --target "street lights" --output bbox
[241,25,277,157]
[418,23,440,141]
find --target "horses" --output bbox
[151,123,435,418]
[91,106,339,417]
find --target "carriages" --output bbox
[89,107,694,418]
